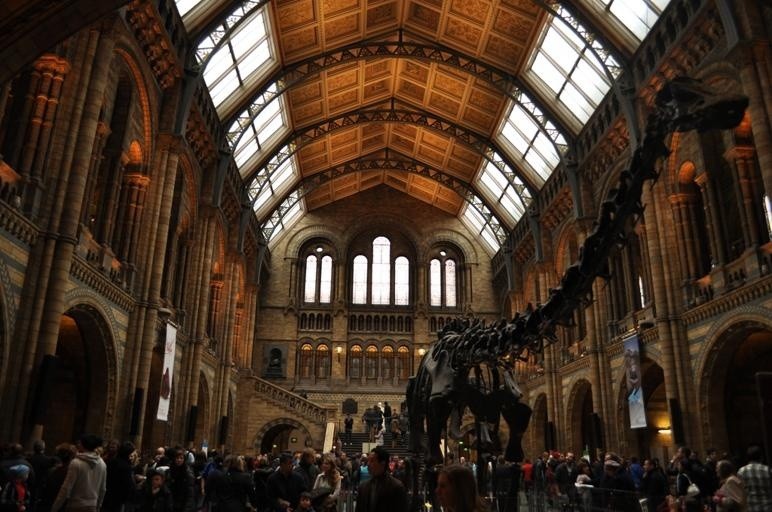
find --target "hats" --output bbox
[9,464,31,479]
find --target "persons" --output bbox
[343,413,354,446]
[360,400,409,434]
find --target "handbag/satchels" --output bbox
[676,473,700,499]
